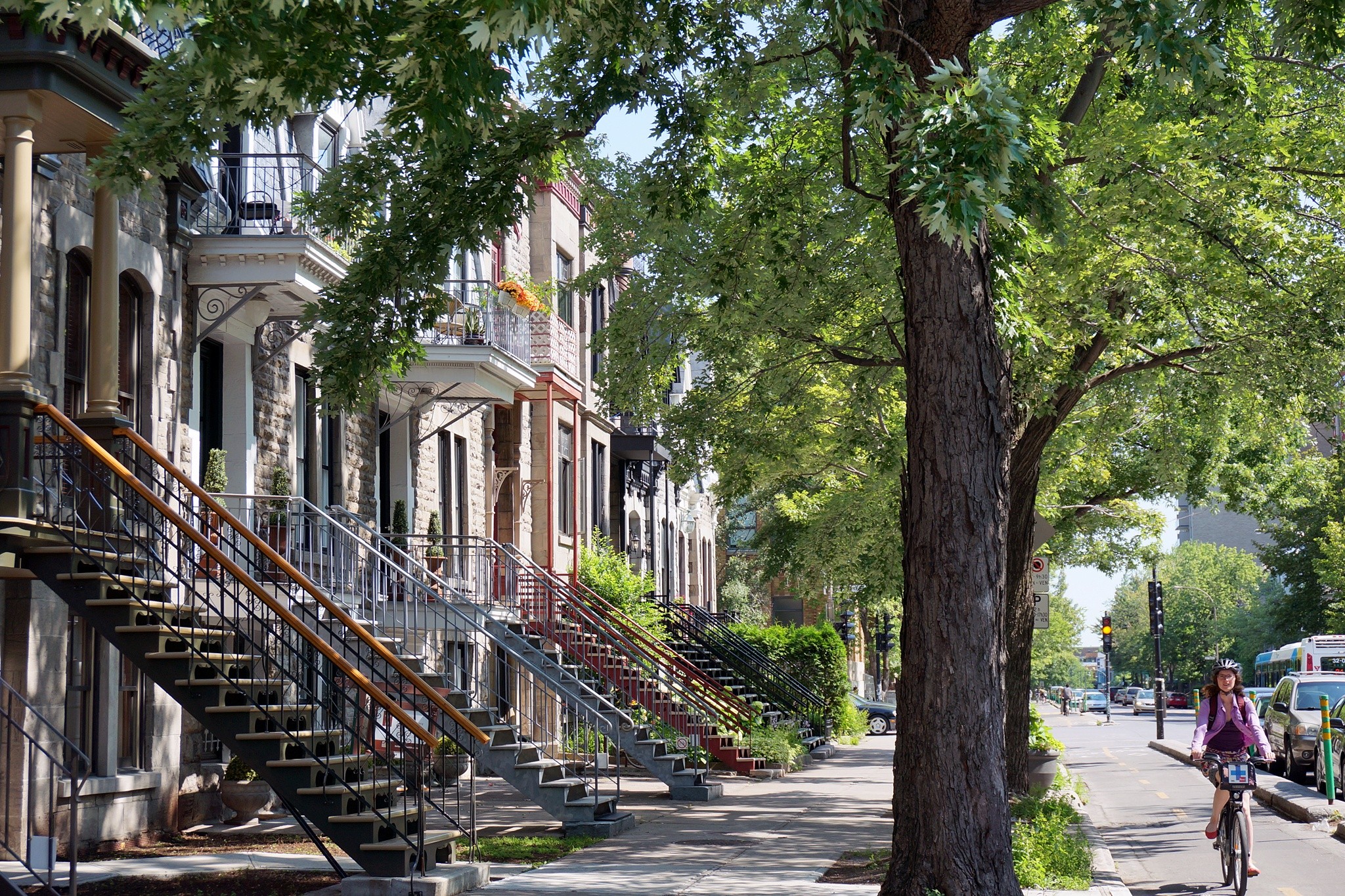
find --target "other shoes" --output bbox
[1205,822,1217,839]
[1069,708,1071,710]
[1247,868,1261,878]
[1063,713,1066,716]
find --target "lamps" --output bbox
[415,388,434,414]
[245,292,271,327]
[627,533,640,555]
[681,513,696,533]
[642,539,651,558]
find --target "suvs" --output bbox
[1263,670,1345,784]
[1121,685,1146,706]
[1109,686,1124,701]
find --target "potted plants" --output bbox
[1028,707,1064,798]
[196,448,295,583]
[339,735,469,796]
[464,306,486,345]
[389,500,448,603]
[221,756,272,825]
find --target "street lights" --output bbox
[1084,670,1091,713]
[1173,585,1219,662]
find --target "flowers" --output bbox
[282,212,333,244]
[497,264,561,316]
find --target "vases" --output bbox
[498,290,517,309]
[512,302,530,318]
[282,221,300,235]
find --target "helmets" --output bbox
[1212,658,1243,674]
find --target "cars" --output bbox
[1114,689,1126,703]
[836,689,897,736]
[1241,686,1335,770]
[1079,692,1107,714]
[1132,689,1167,715]
[1050,686,1099,705]
[1313,694,1345,803]
[1165,691,1188,709]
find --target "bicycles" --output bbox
[1189,752,1276,896]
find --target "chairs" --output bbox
[220,158,280,236]
[1298,694,1314,708]
[428,291,468,345]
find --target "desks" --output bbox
[438,302,482,345]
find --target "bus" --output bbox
[1254,634,1345,701]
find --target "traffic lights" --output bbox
[875,633,886,653]
[1103,616,1112,653]
[833,621,845,641]
[884,624,896,653]
[841,611,855,645]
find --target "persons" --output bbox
[1191,658,1276,877]
[1029,687,1047,704]
[1060,684,1073,716]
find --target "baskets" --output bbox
[1220,761,1257,790]
